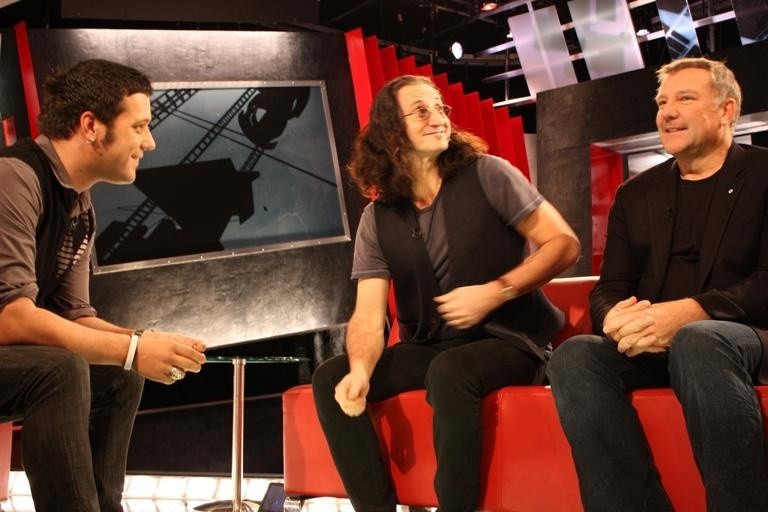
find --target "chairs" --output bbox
[1,420,16,502]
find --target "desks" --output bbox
[202,355,308,511]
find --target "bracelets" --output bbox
[123,335,138,371]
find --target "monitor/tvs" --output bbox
[90,79,352,277]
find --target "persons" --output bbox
[544,57,768,512]
[311,75,582,512]
[0,59,208,512]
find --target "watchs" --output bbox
[497,279,517,301]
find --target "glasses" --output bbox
[399,104,453,121]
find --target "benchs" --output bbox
[284,277,767,512]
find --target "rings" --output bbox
[169,368,182,381]
